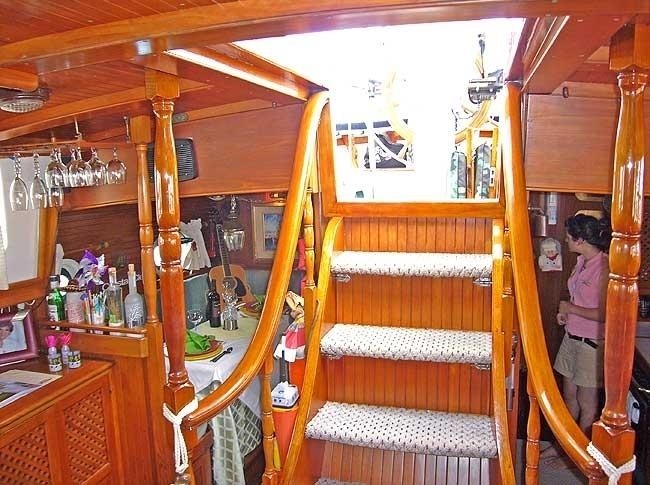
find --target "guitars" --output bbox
[207,207,256,314]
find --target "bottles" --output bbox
[61,344,70,364]
[125,264,142,328]
[66,286,86,333]
[46,276,62,320]
[48,345,59,364]
[208,278,221,327]
[105,268,122,326]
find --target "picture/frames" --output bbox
[251,205,299,263]
[0,312,40,364]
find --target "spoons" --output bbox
[210,346,234,363]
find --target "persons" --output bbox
[540,212,610,468]
[539,241,561,271]
[0,322,14,354]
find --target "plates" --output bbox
[185,344,224,361]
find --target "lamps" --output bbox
[2,81,52,113]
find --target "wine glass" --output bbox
[8,151,29,213]
[88,147,107,188]
[46,148,70,190]
[46,188,64,209]
[186,308,202,332]
[106,146,126,186]
[29,153,48,211]
[66,146,94,189]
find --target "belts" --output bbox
[567,332,598,349]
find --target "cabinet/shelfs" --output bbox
[0,363,124,485]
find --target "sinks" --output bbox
[635,337,650,366]
